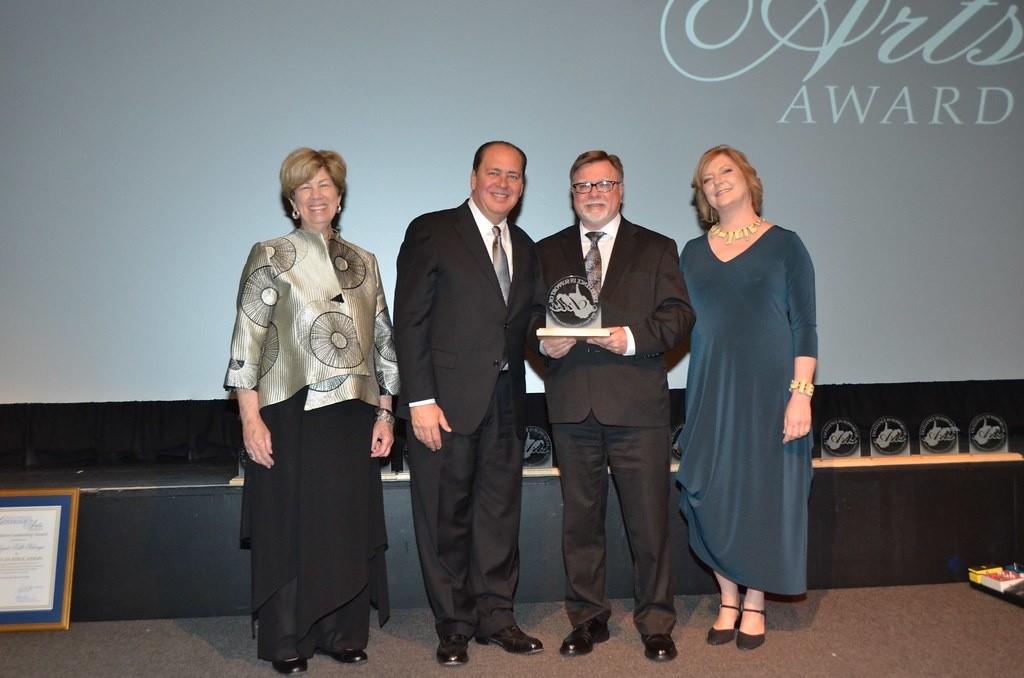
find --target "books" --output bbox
[536,327,611,342]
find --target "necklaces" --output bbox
[711,217,763,244]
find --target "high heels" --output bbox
[738,603,767,649]
[707,602,743,646]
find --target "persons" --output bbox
[223,148,398,673]
[525,151,696,668]
[394,141,543,665]
[674,144,817,650]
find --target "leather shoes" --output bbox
[437,630,469,666]
[559,616,609,657]
[475,622,542,654]
[272,656,308,675]
[316,646,369,664]
[640,627,675,663]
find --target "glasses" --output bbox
[572,179,622,195]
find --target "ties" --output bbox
[491,224,511,371]
[584,231,606,302]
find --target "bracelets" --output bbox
[789,379,815,398]
[375,410,395,425]
